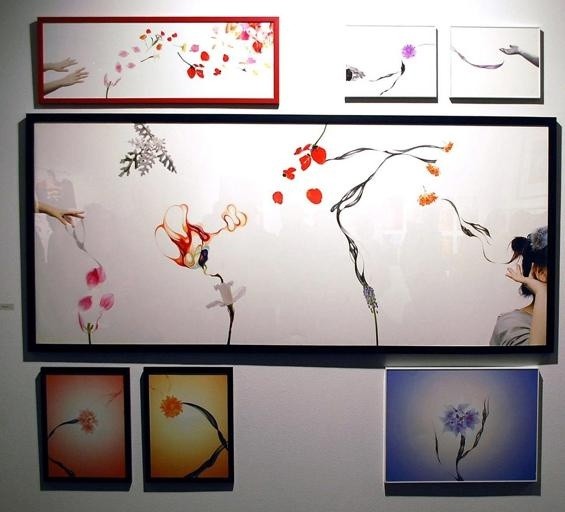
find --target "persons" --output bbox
[35,199,85,225]
[499,43,539,69]
[43,57,91,97]
[487,227,547,346]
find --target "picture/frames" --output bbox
[446,25,542,99]
[140,367,234,486]
[35,366,133,485]
[16,113,560,357]
[344,25,438,98]
[382,368,540,484]
[36,15,280,106]
